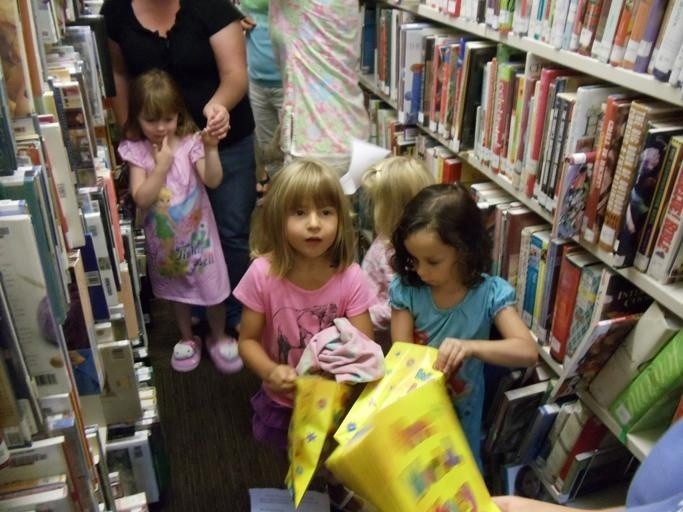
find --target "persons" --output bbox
[230,156,380,487]
[90,1,258,342]
[261,0,375,175]
[113,68,249,377]
[488,415,681,511]
[390,183,540,474]
[354,155,436,333]
[225,2,292,156]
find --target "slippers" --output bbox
[204,336,243,376]
[170,336,203,373]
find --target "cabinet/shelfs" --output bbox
[365,1,680,511]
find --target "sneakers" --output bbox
[255,173,270,207]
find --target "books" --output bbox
[376,1,680,284]
[481,224,680,504]
[0,1,170,511]
